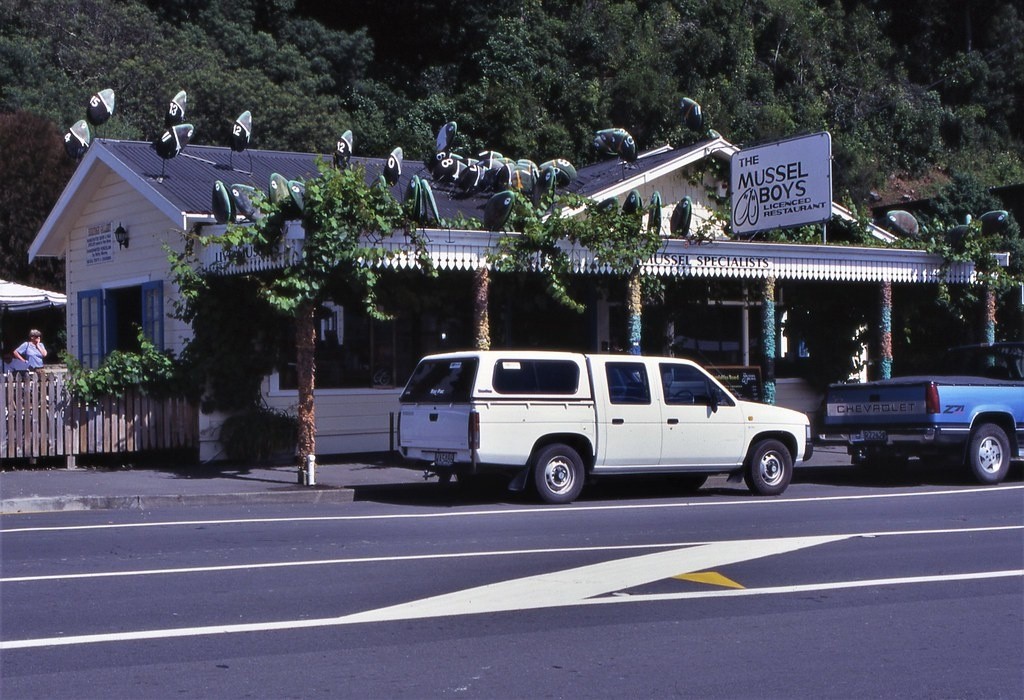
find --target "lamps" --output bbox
[114,220,129,251]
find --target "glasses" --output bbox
[31,335,41,338]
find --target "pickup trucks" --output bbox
[390,349,815,505]
[814,337,1023,487]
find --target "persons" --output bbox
[13,329,48,372]
[0,348,31,376]
